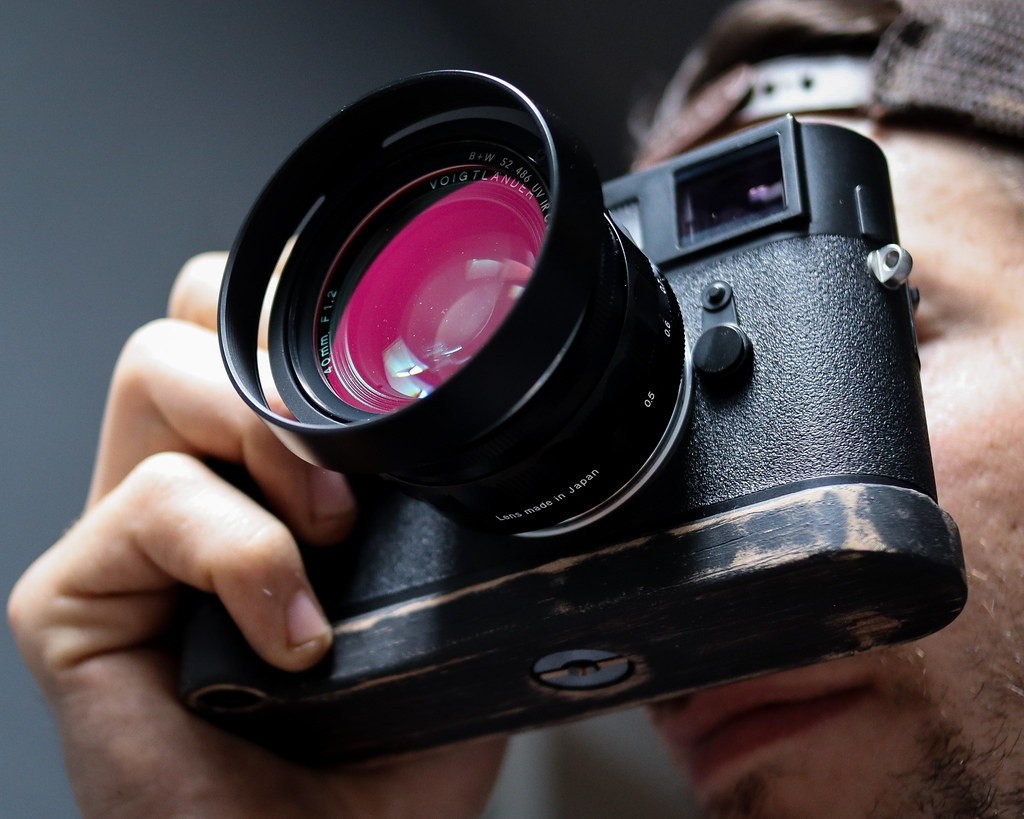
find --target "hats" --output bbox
[631,0,1024,141]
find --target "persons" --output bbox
[6,0,1024,819]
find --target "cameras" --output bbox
[177,70,970,773]
